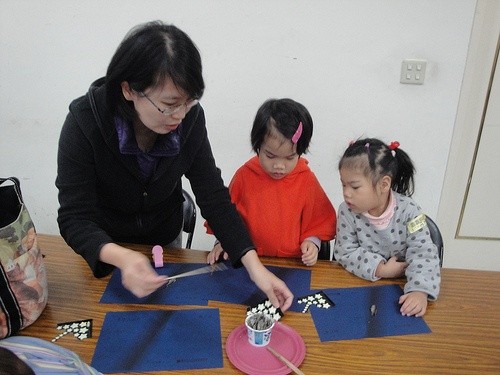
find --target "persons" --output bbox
[333,138,442,317]
[204,99,336,266]
[55,20,294,312]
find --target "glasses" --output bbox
[140,91,199,116]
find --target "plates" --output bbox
[225,321,306,375]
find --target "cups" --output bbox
[245,314,275,347]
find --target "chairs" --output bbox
[182,189,196,249]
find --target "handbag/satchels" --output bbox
[0,176,48,340]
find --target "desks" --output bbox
[16,233,500,375]
